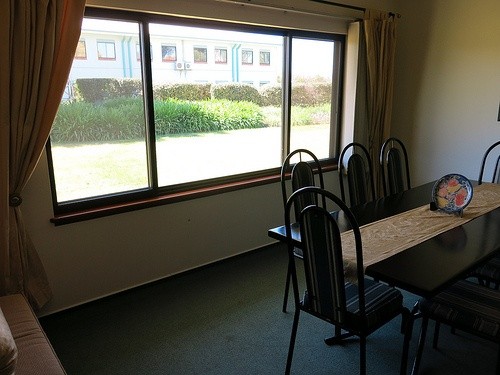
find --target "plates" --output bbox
[432,174,473,214]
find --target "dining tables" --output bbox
[268,174,500,375]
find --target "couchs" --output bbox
[0,292,67,375]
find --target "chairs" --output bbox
[280,138,500,375]
